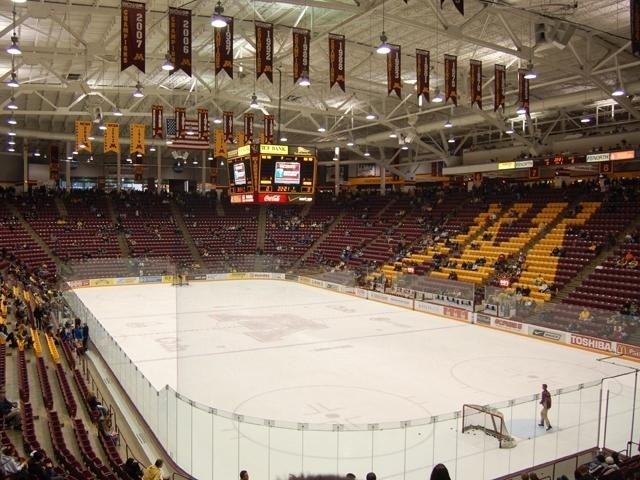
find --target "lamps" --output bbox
[7,0,625,164]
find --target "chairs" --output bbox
[0,195,640,480]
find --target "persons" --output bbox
[239,470,249,480]
[0,175,640,277]
[430,463,451,480]
[354,276,639,343]
[0,278,164,480]
[539,384,553,431]
[521,442,640,480]
[346,472,376,480]
